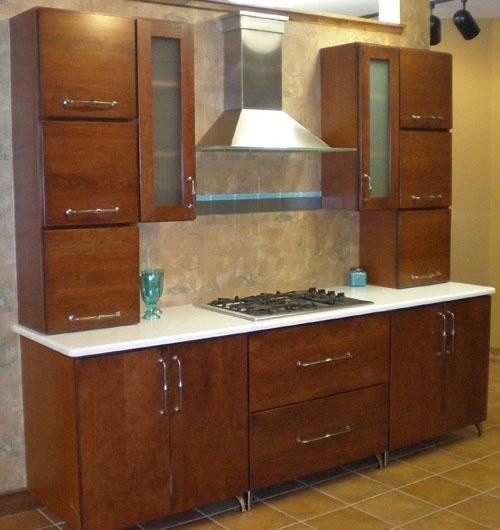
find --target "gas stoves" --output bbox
[201,287,373,321]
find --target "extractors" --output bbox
[194,8,358,153]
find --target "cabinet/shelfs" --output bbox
[248,312,391,493]
[392,296,491,452]
[135,19,200,223]
[18,337,249,526]
[10,6,142,334]
[320,42,399,212]
[360,47,453,287]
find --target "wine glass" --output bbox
[140,269,165,321]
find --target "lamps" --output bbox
[429,1,481,46]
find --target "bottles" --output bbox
[347,267,368,287]
